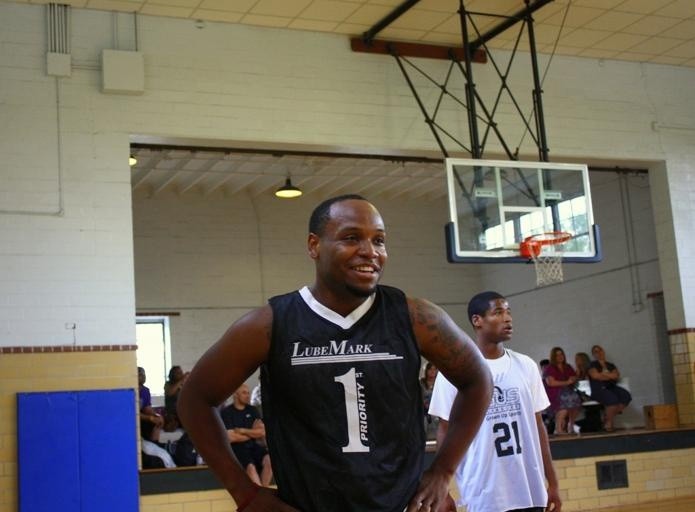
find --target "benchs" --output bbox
[542,370,629,436]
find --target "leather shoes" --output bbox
[553,427,578,437]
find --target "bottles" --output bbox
[196,454,206,466]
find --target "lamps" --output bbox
[273,163,307,200]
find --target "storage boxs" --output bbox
[642,402,681,430]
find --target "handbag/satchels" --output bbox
[568,384,592,403]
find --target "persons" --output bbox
[428,291,562,512]
[176,195,495,512]
